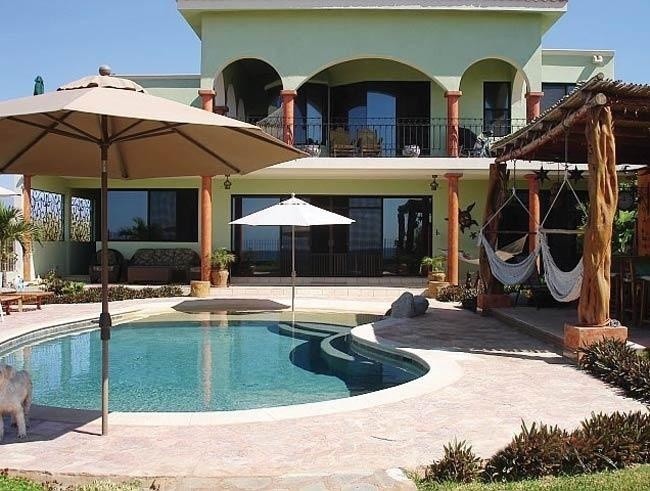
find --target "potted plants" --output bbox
[302,139,322,156]
[210,248,239,287]
[418,251,449,282]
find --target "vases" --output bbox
[402,144,421,157]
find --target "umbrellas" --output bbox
[0,66,310,436]
[228,192,356,310]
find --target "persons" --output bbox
[472,128,494,158]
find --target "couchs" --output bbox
[128,248,199,282]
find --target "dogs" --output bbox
[0,360,34,440]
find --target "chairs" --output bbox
[611,255,650,327]
[359,128,383,157]
[329,127,361,157]
[3,291,54,310]
[89,249,124,283]
[0,296,23,315]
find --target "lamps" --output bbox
[224,175,231,190]
[430,175,439,191]
